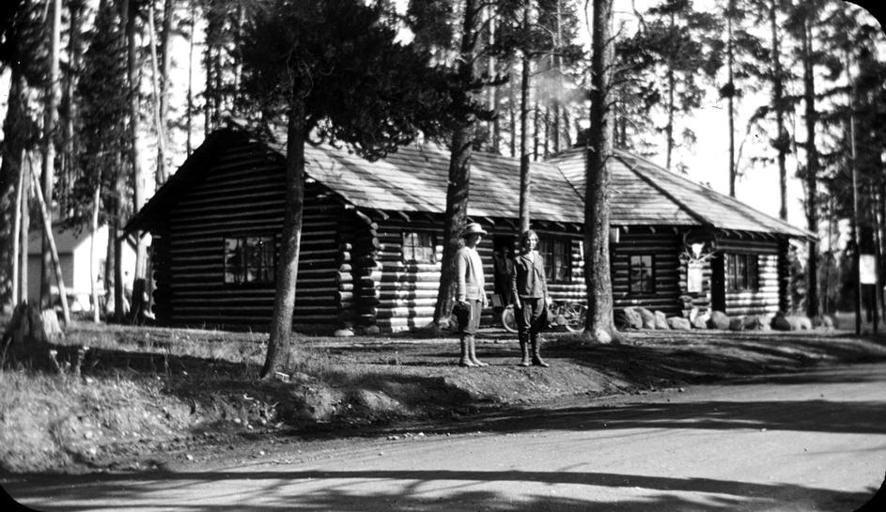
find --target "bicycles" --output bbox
[501,292,588,333]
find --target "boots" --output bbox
[518,332,529,367]
[530,332,549,368]
[469,338,488,367]
[459,336,479,368]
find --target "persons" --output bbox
[493,245,514,300]
[452,223,489,368]
[508,230,551,367]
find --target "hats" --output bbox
[461,223,488,239]
